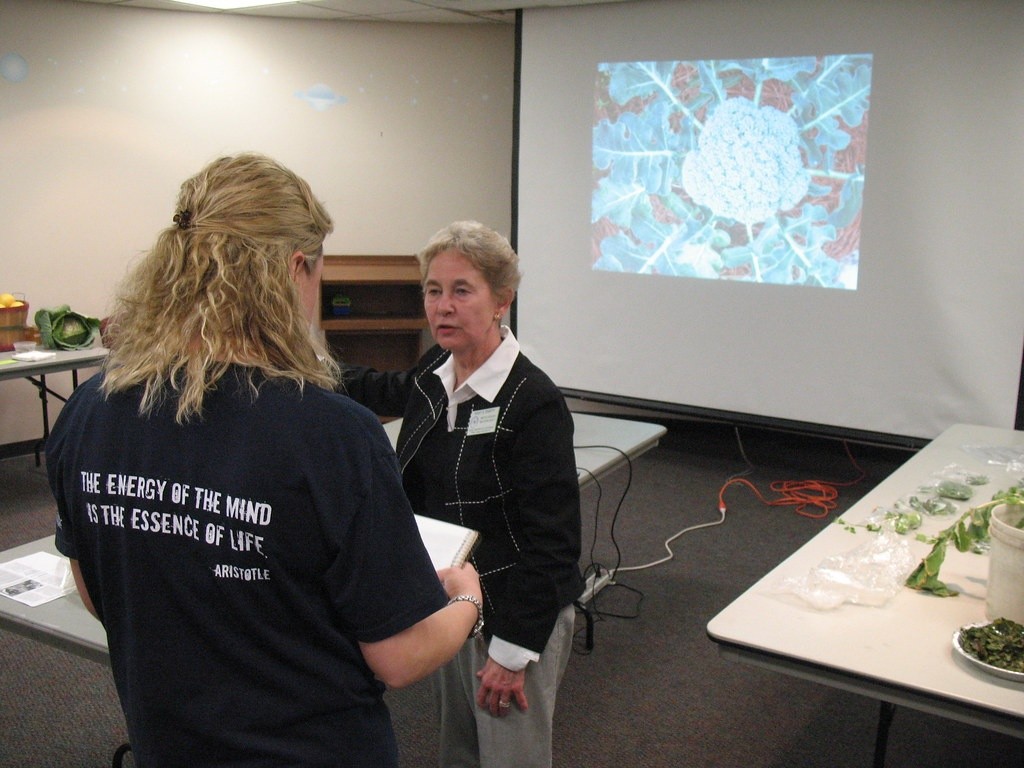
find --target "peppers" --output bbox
[959,617,1024,672]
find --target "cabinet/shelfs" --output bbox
[316,254,428,423]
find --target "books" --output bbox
[414,513,481,572]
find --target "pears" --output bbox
[0,293,25,308]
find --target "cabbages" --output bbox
[35,304,101,351]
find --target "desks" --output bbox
[0,411,667,768]
[705,422,1024,768]
[0,345,111,467]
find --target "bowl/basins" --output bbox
[13,341,37,352]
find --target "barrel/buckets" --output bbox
[0,292,30,351]
[982,499,1024,626]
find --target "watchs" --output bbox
[447,595,484,641]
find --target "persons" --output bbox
[332,220,584,768]
[45,153,485,768]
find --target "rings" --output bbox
[499,700,511,707]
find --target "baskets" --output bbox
[0,300,30,351]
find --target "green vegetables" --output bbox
[836,476,1024,597]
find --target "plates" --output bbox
[953,620,1024,682]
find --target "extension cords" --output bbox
[575,569,613,611]
